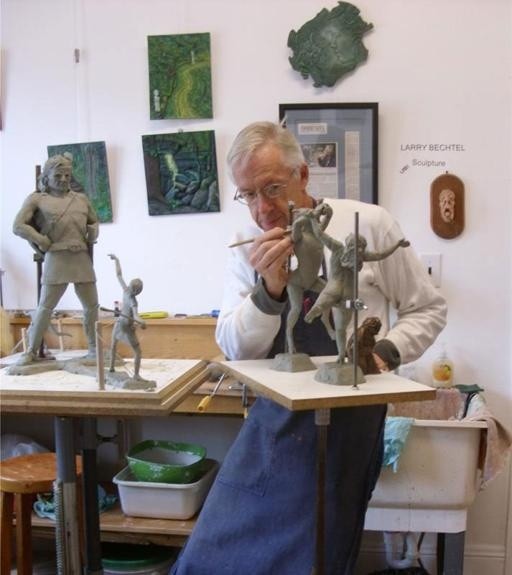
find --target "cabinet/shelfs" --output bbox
[1,315,256,555]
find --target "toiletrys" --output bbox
[431,347,453,388]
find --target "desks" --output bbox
[216,355,438,575]
[0,357,212,574]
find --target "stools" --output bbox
[0,452,84,575]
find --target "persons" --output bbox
[12,152,99,366]
[108,254,148,380]
[167,120,448,575]
[304,211,411,365]
[282,204,337,350]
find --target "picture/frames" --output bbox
[278,102,379,204]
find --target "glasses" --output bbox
[234,166,299,205]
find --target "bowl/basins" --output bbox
[101,556,174,575]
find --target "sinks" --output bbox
[363,387,488,533]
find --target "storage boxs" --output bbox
[112,458,222,520]
